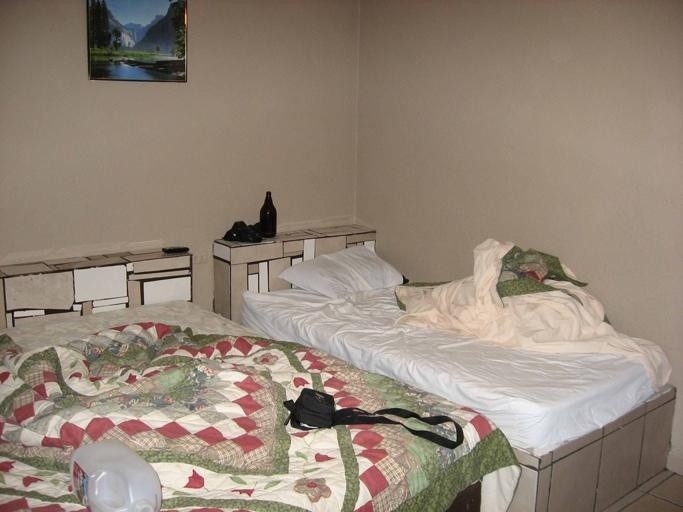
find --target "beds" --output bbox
[0,246,501,510]
[210,227,676,508]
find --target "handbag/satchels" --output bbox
[280,384,338,431]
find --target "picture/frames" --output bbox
[87,0,188,82]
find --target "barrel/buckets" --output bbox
[69,439,162,512]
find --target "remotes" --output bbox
[162,247,189,254]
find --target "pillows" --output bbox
[278,243,409,298]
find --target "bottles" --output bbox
[258,190,276,237]
